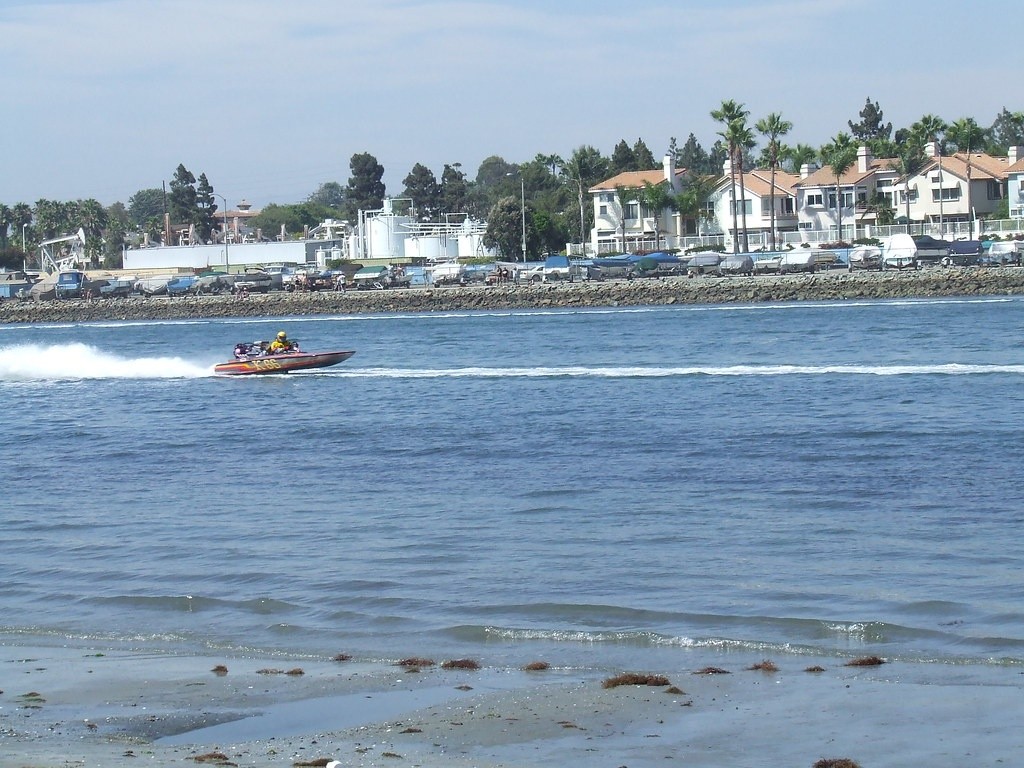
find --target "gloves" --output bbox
[284,346,290,350]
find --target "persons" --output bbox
[271,332,300,355]
[496,265,518,287]
[285,275,317,295]
[59,289,70,300]
[80,287,93,304]
[333,272,346,293]
[214,284,249,300]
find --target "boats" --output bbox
[31,265,335,297]
[884,232,919,271]
[353,257,840,291]
[847,245,884,272]
[988,241,1022,267]
[942,241,986,269]
[213,339,356,377]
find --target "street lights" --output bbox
[506,173,527,264]
[208,193,230,273]
[22,223,29,273]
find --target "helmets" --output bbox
[277,332,286,343]
